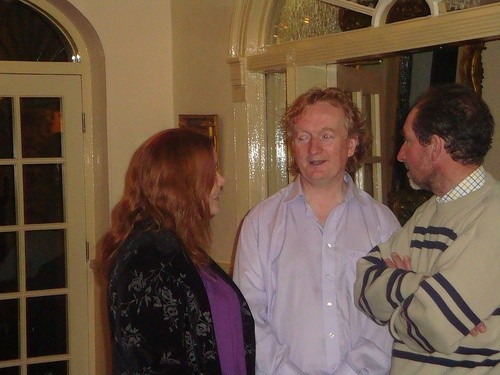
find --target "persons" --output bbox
[352,84,500,375]
[90,128,257,374]
[231,87,401,375]
